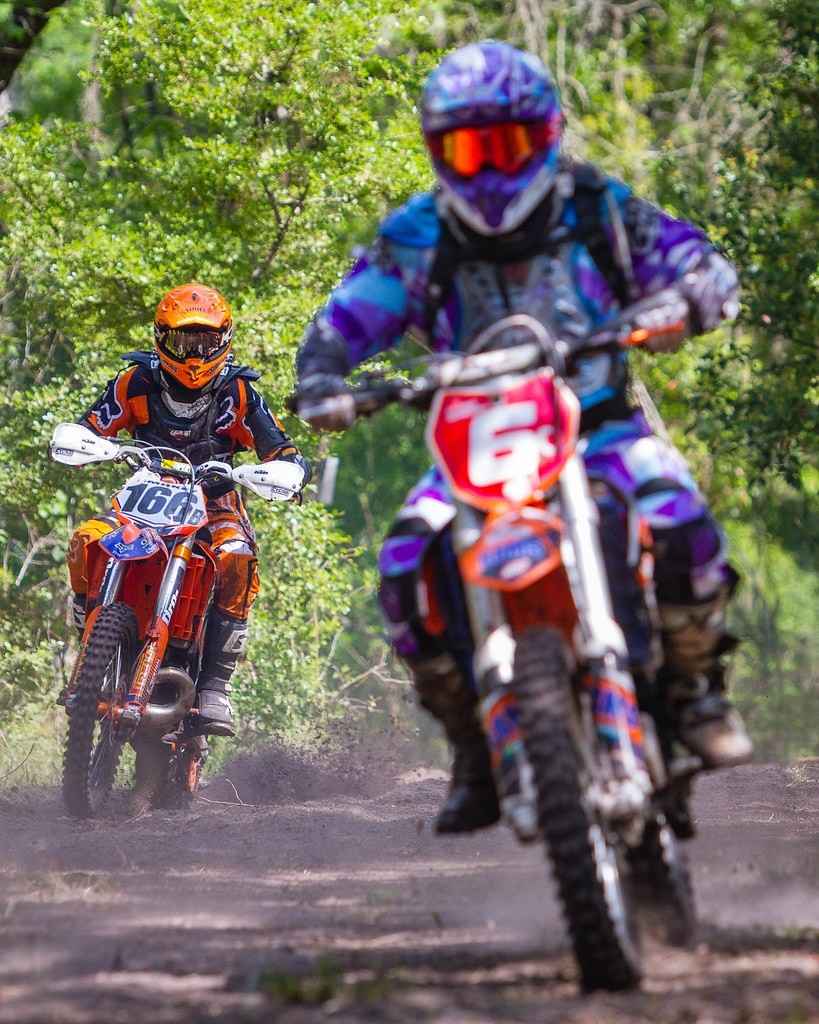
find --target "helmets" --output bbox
[153,283,234,389]
[420,38,565,236]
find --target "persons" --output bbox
[293,42,754,840]
[47,283,312,737]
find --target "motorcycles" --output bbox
[45,420,308,820]
[297,316,730,991]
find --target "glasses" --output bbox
[427,121,553,176]
[154,325,223,364]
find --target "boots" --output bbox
[657,584,753,767]
[400,637,501,835]
[72,593,85,636]
[192,604,247,736]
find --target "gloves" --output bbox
[631,289,687,356]
[298,373,357,431]
